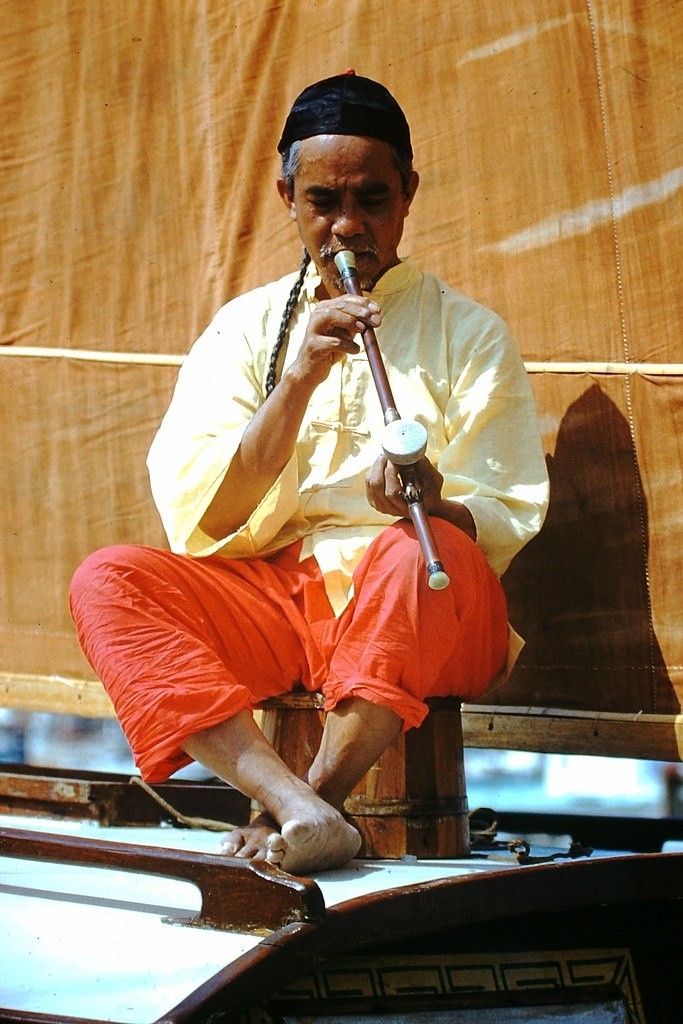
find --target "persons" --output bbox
[65,69,553,877]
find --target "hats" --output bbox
[277,70,411,156]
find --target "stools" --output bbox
[246,690,475,860]
